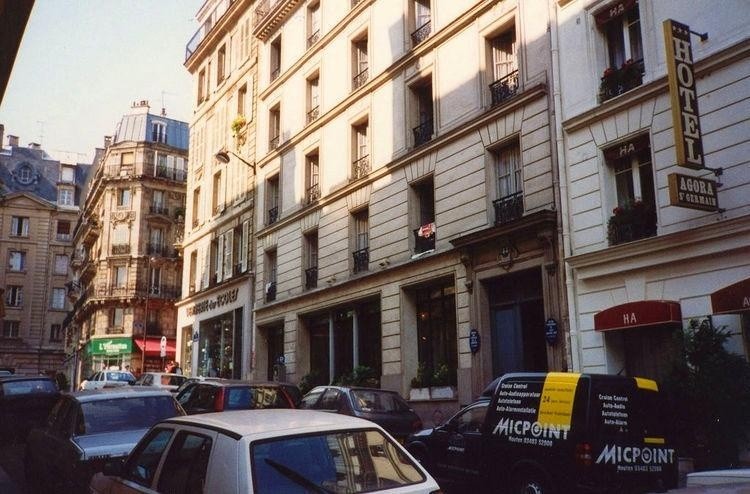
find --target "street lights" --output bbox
[141,253,164,374]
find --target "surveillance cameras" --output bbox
[326,277,336,284]
[378,258,390,268]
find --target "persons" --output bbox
[171,362,183,384]
[165,359,176,372]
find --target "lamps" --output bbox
[217,150,256,174]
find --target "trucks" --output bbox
[405,372,679,494]
